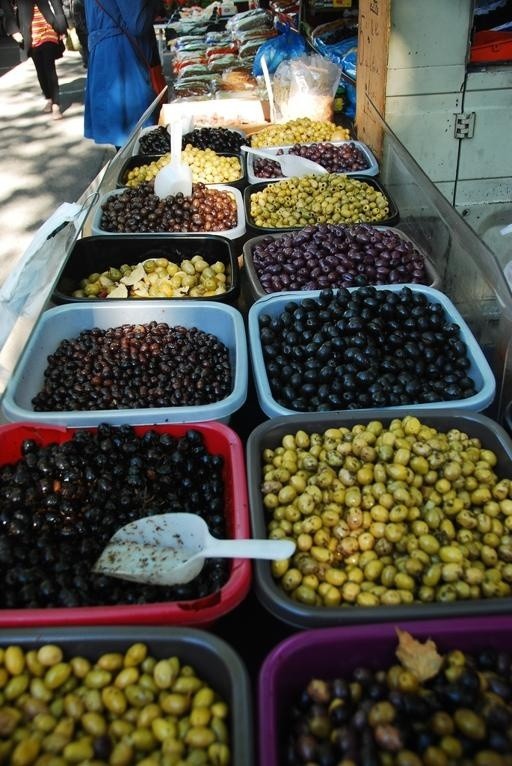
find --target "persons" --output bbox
[0,0,168,152]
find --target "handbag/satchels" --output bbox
[149,64,168,103]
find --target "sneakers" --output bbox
[44,102,63,119]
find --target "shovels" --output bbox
[90,512,298,585]
[240,145,330,177]
[154,121,193,200]
